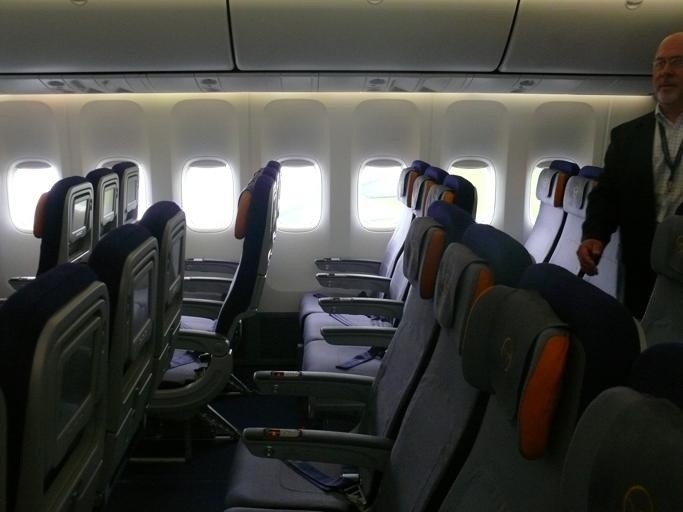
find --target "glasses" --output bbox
[652,58,682,69]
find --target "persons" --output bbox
[576,31,683,323]
